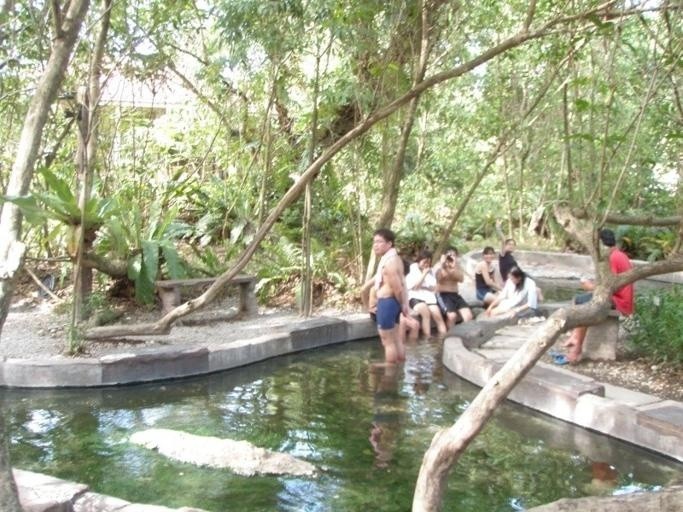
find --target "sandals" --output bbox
[546,348,572,366]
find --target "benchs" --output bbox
[575,308,619,362]
[153,273,258,317]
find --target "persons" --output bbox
[559,230,633,365]
[361,229,544,364]
